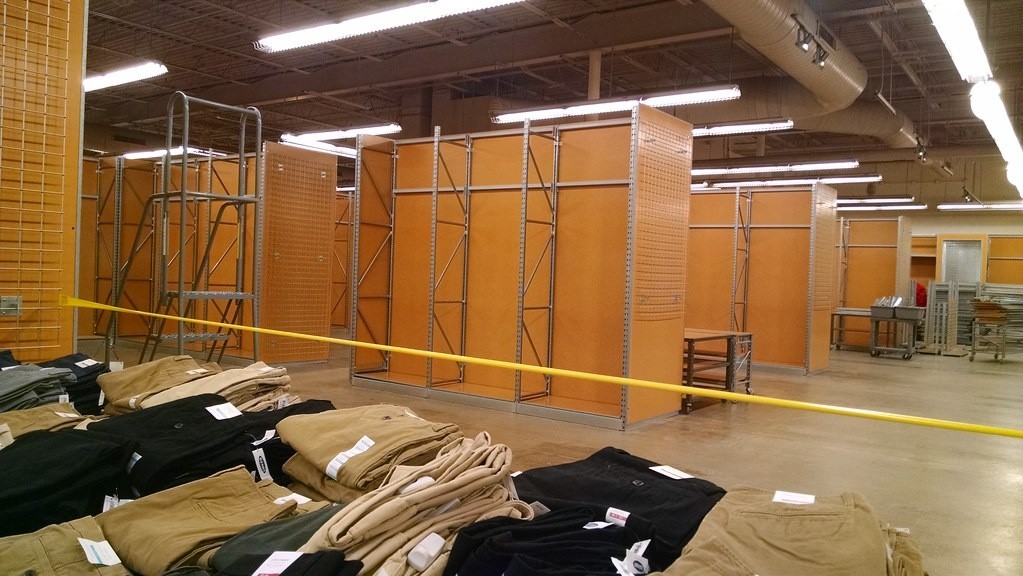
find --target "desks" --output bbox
[830,310,918,363]
[684,326,753,413]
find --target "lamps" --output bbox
[794,28,829,70]
